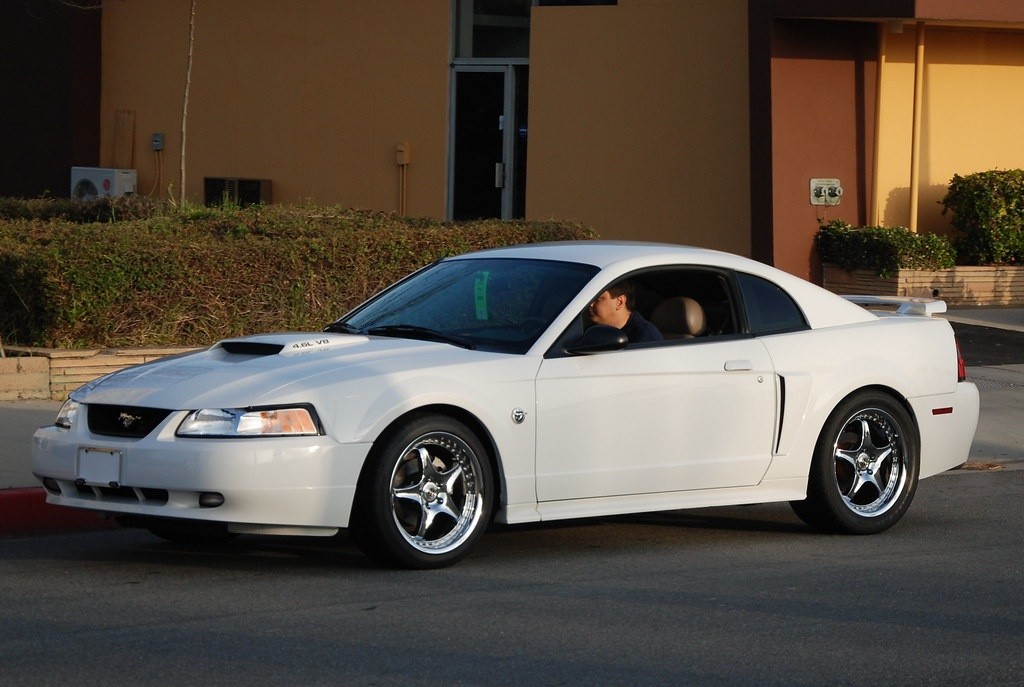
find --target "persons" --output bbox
[589,278,663,344]
[539,269,596,333]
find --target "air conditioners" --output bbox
[70,167,135,199]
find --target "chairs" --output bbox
[649,297,704,340]
[538,278,583,346]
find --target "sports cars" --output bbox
[32,239,982,571]
